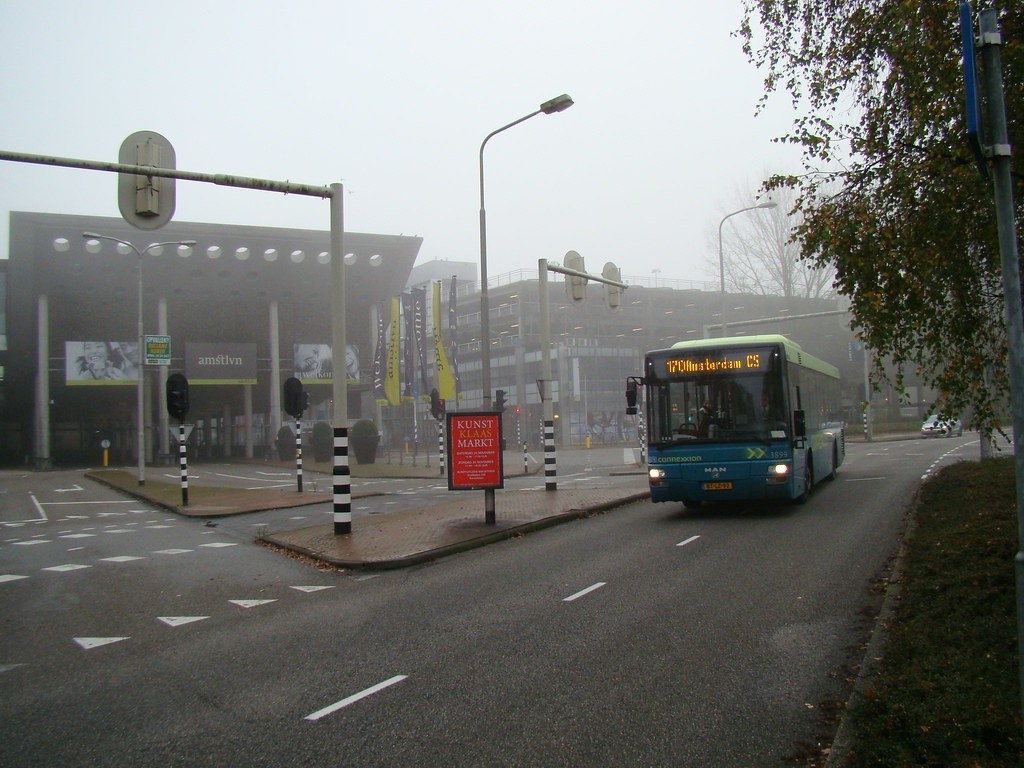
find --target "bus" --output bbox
[623,332,846,511]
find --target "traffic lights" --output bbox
[305,391,310,410]
[514,406,520,415]
[496,389,507,414]
[430,390,446,420]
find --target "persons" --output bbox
[698,399,726,434]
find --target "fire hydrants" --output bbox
[584,430,593,448]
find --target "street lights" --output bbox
[478,92,577,527]
[81,231,196,484]
[718,202,778,340]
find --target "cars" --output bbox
[920,413,962,439]
